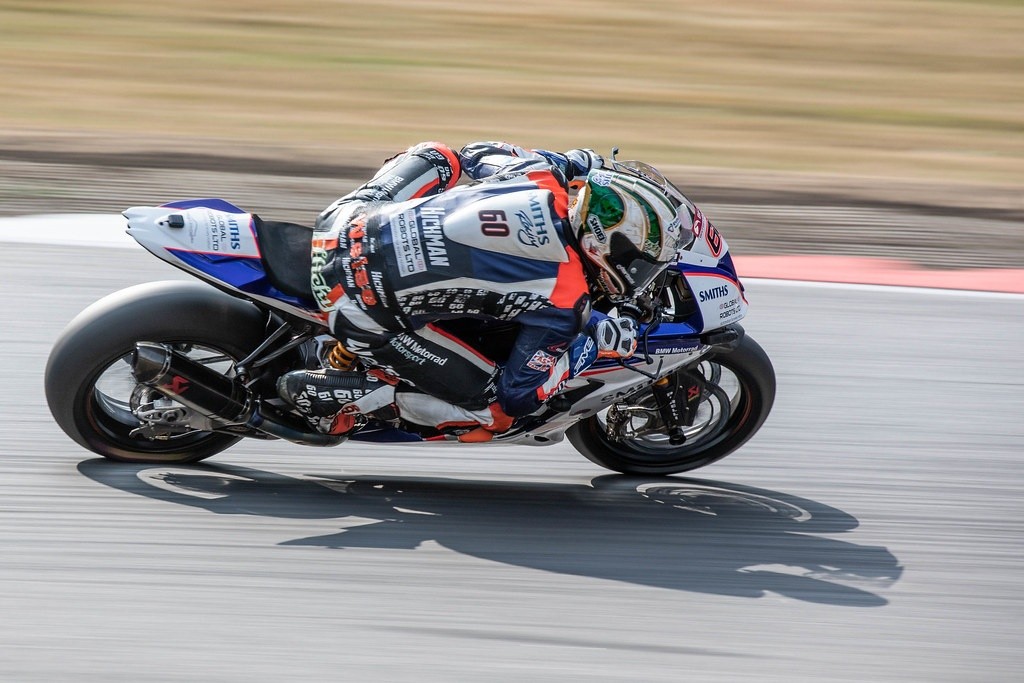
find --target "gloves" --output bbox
[557,147,605,181]
[587,315,639,359]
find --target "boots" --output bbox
[276,366,415,437]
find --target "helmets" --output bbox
[568,168,679,305]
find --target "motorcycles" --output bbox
[45,146,775,478]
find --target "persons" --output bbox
[277,140,682,437]
